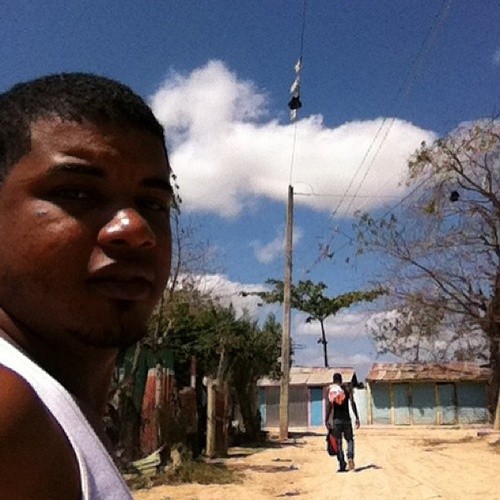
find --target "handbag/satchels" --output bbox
[328,430,338,457]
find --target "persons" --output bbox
[1,71,174,499]
[324,372,362,471]
